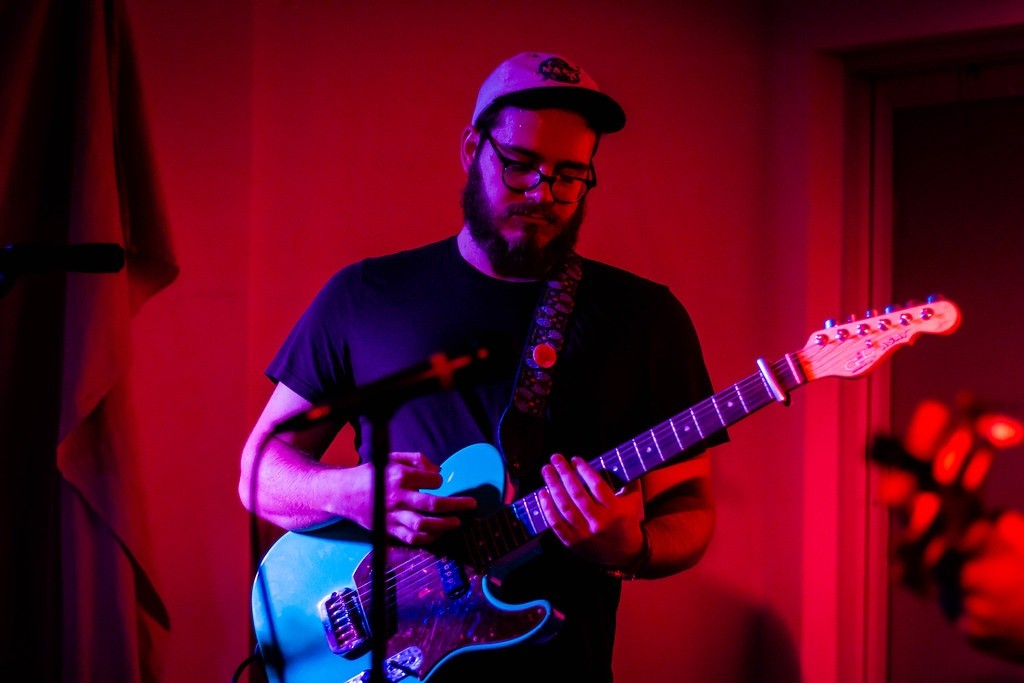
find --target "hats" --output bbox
[471,52,626,133]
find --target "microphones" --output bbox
[285,336,504,428]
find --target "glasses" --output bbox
[485,131,597,204]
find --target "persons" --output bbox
[237,49,730,683]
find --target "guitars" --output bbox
[247,287,963,682]
